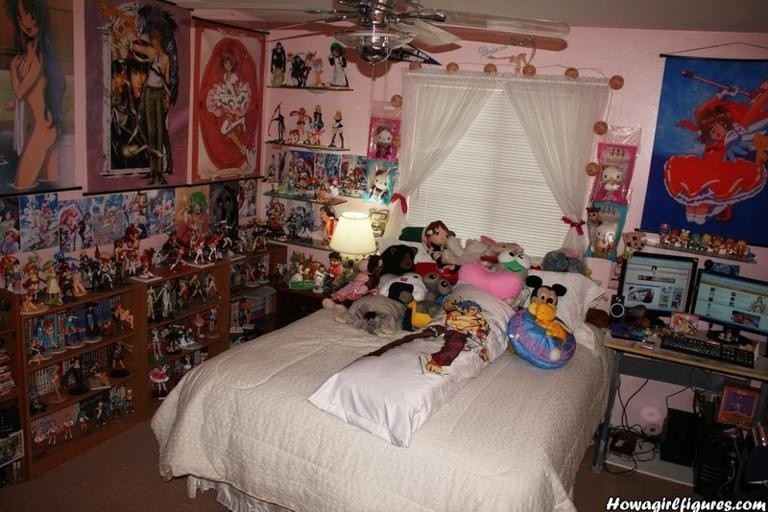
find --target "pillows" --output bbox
[379,239,435,265]
[527,269,603,351]
[308,283,515,453]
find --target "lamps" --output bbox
[328,209,377,256]
[334,26,414,63]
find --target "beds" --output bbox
[148,223,620,512]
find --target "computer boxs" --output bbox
[692,399,745,495]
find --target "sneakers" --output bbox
[147,174,156,185]
[240,117,246,134]
[158,174,168,185]
[244,149,251,165]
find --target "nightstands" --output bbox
[274,281,330,322]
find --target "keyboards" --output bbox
[659,333,754,369]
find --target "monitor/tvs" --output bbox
[690,268,768,347]
[617,251,699,328]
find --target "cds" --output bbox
[644,423,663,435]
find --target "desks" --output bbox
[597,329,767,492]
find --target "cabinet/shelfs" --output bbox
[261,78,358,254]
[0,242,287,489]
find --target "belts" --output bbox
[147,85,165,90]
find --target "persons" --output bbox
[0,0,759,490]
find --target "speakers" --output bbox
[609,295,625,325]
[658,409,701,466]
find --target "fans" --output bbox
[170,0,574,39]
